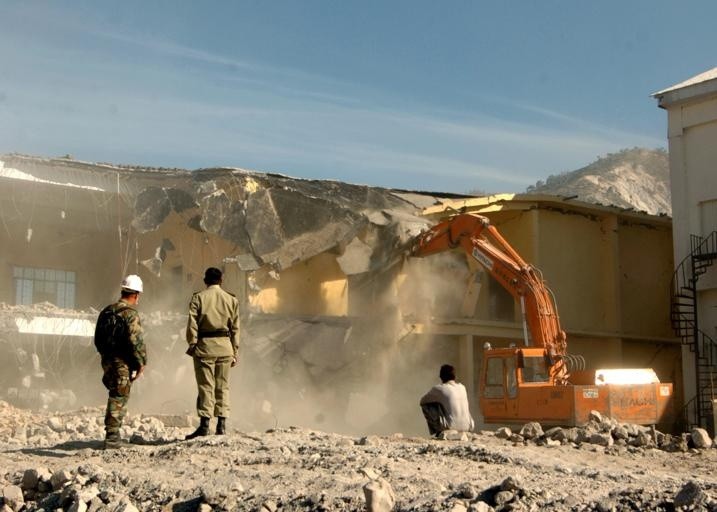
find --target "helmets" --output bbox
[120,273,144,293]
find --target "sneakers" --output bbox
[103,430,135,448]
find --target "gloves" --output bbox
[183,344,196,356]
[230,354,241,367]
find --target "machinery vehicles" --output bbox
[400,212,676,428]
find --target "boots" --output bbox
[183,414,211,442]
[215,417,227,436]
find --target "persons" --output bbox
[92,273,147,448]
[417,364,473,439]
[183,267,241,440]
